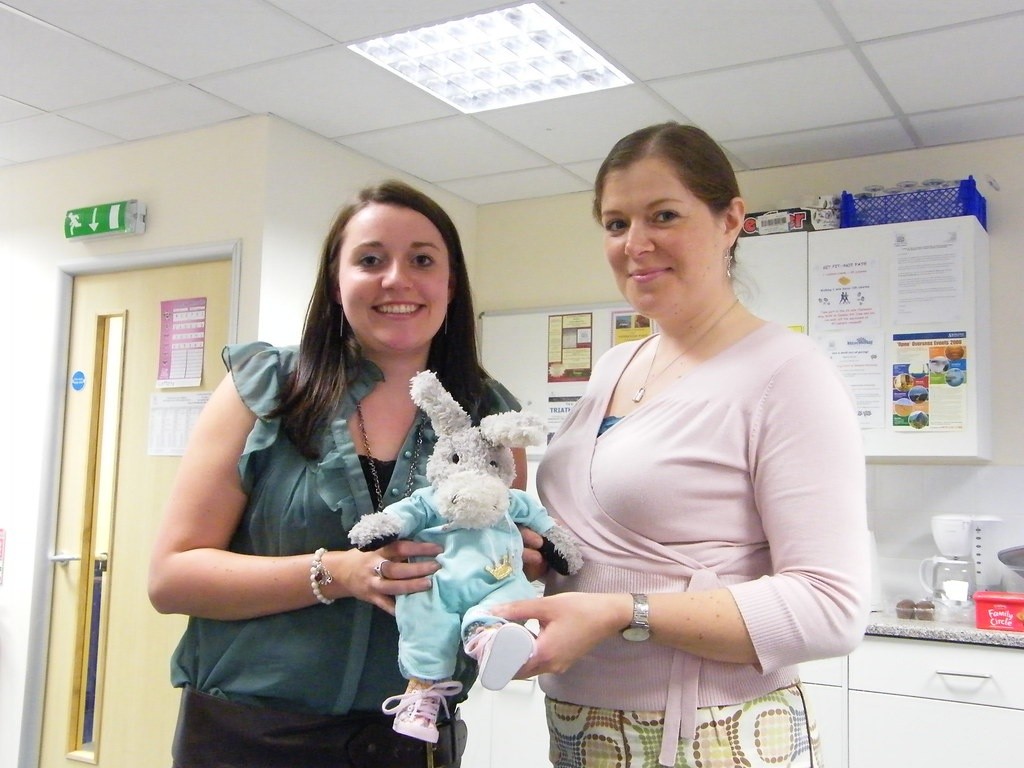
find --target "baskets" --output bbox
[839,174,987,231]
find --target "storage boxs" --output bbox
[973,590,1024,632]
[840,175,988,232]
[737,207,839,237]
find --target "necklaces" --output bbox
[355,402,430,513]
[631,297,739,404]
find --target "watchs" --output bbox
[621,593,654,642]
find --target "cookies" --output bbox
[895,599,934,621]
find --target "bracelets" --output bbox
[309,548,336,604]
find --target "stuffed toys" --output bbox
[345,368,587,742]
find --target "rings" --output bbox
[373,560,390,579]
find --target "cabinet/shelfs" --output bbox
[455,619,553,768]
[848,634,1024,768]
[731,214,993,464]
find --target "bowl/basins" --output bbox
[894,606,916,619]
[915,608,934,621]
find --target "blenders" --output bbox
[920,516,979,624]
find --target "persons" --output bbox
[147,179,528,768]
[509,121,873,768]
